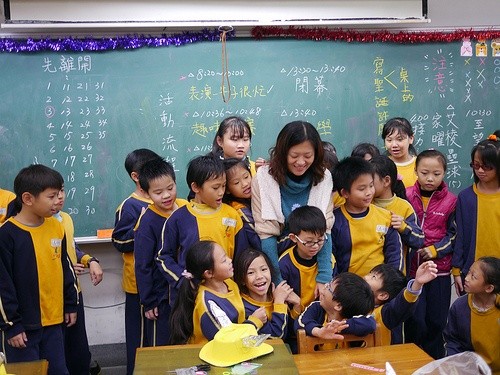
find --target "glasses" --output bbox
[294,233,328,247]
[326,282,339,300]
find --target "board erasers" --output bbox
[96,228,114,239]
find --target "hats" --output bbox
[198,323,273,368]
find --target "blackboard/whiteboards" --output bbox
[1,23,500,243]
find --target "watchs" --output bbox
[87,256,100,268]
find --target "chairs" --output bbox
[296,329,375,354]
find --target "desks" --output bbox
[292,343,436,375]
[4,359,49,375]
[133,339,300,375]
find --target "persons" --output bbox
[133,157,190,346]
[0,164,105,375]
[405,149,459,361]
[155,155,251,310]
[380,117,419,189]
[210,114,404,308]
[176,240,268,345]
[361,260,438,347]
[450,131,500,297]
[278,205,338,356]
[112,147,165,375]
[368,155,426,346]
[294,271,377,352]
[234,246,302,341]
[443,256,500,375]
